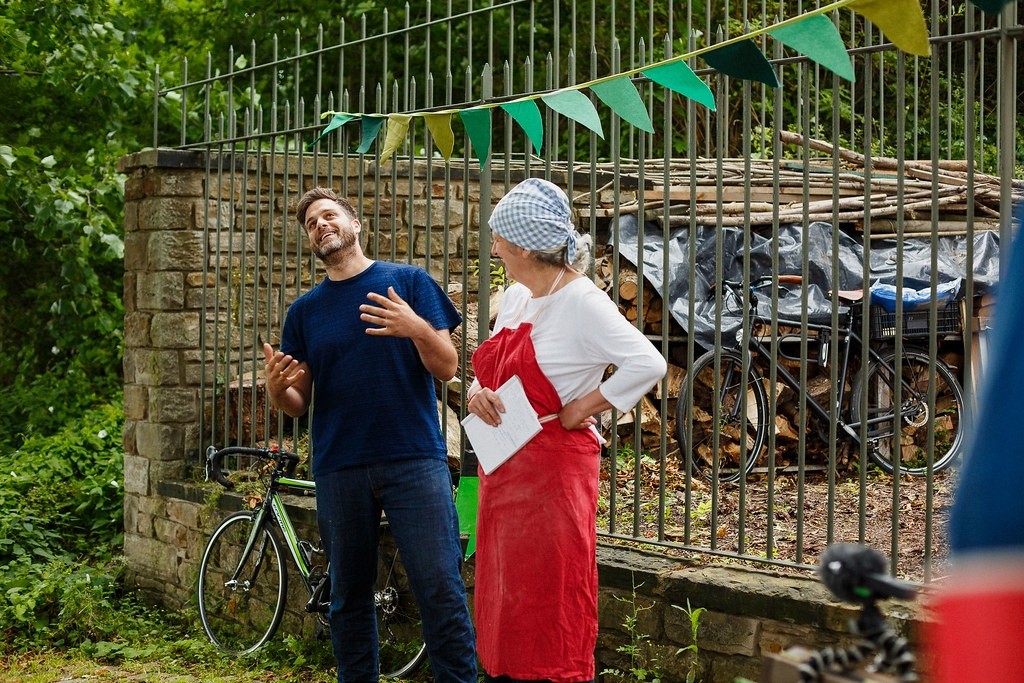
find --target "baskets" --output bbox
[869,299,959,341]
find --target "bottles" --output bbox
[455,438,480,536]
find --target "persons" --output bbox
[263,187,481,683]
[475,180,667,683]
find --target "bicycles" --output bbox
[201,444,432,682]
[675,274,967,487]
